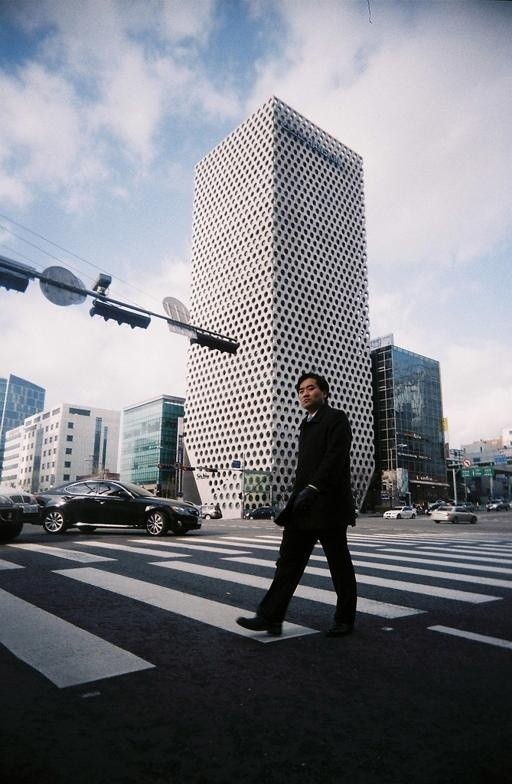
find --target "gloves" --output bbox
[295,486,318,515]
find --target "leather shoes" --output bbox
[327,623,355,639]
[236,617,282,636]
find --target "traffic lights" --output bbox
[182,467,195,471]
[205,468,217,472]
[158,465,164,469]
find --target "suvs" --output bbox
[197,504,222,519]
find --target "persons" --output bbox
[237,373,358,638]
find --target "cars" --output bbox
[244,506,274,520]
[0,494,26,544]
[382,496,512,525]
[33,479,203,537]
[0,486,41,522]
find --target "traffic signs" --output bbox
[461,467,495,478]
